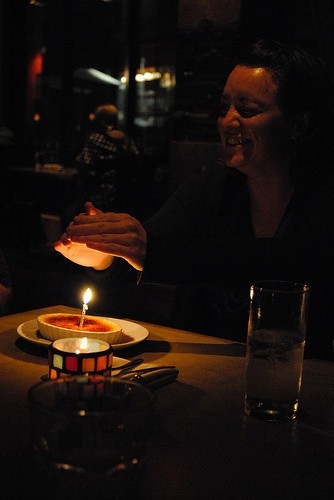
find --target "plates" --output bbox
[17,312,152,348]
[36,161,68,174]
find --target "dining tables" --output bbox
[0,305,334,500]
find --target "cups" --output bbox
[242,279,309,424]
[27,376,154,500]
[43,337,114,383]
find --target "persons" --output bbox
[51,40,334,363]
[78,104,141,219]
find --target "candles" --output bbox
[80,288,93,327]
